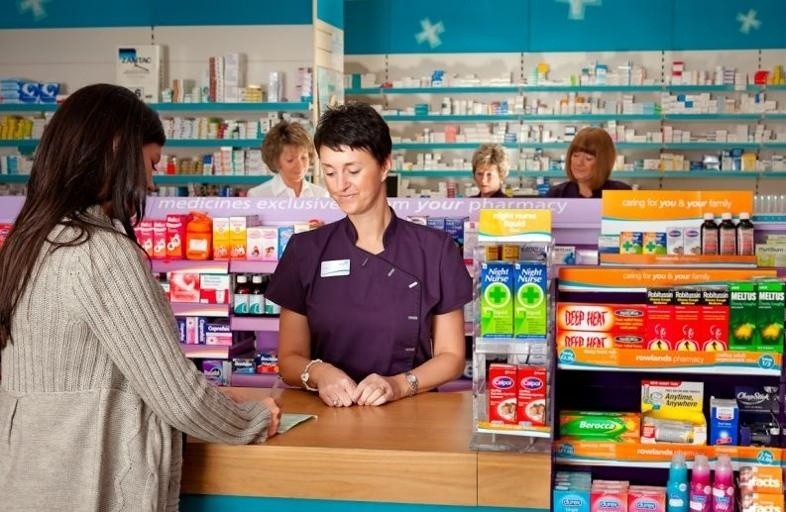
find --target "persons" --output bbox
[0,83,281,512]
[244,121,329,199]
[264,103,472,407]
[542,127,633,200]
[465,143,510,198]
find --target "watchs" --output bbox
[402,370,420,398]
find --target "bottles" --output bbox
[701,213,719,256]
[689,455,710,511]
[711,454,737,512]
[737,213,756,255]
[233,272,252,318]
[265,301,282,317]
[250,275,266,317]
[718,213,738,255]
[667,453,689,512]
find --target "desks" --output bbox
[179,361,550,512]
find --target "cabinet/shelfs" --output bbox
[343,85,781,182]
[553,248,776,512]
[0,102,316,196]
[149,259,287,388]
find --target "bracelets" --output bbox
[298,358,321,392]
